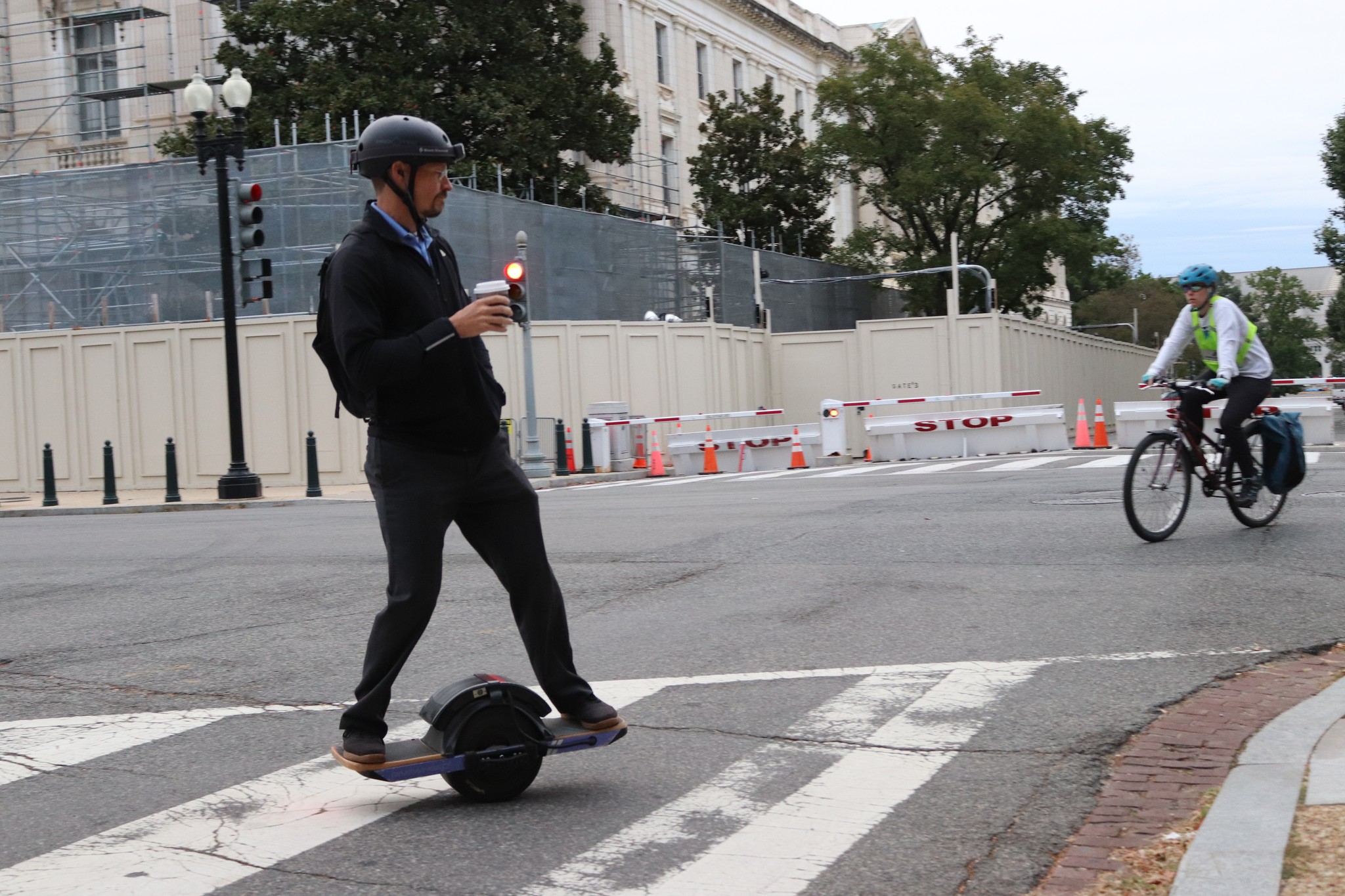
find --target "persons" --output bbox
[325,117,621,764]
[1141,262,1274,508]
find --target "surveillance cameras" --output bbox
[760,269,770,279]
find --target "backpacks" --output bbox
[311,229,391,420]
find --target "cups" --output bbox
[473,280,511,328]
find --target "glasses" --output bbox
[1181,285,1214,292]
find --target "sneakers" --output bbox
[1173,447,1205,471]
[1234,474,1262,507]
[560,694,619,730]
[342,729,385,763]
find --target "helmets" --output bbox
[350,115,467,177]
[1179,263,1218,312]
